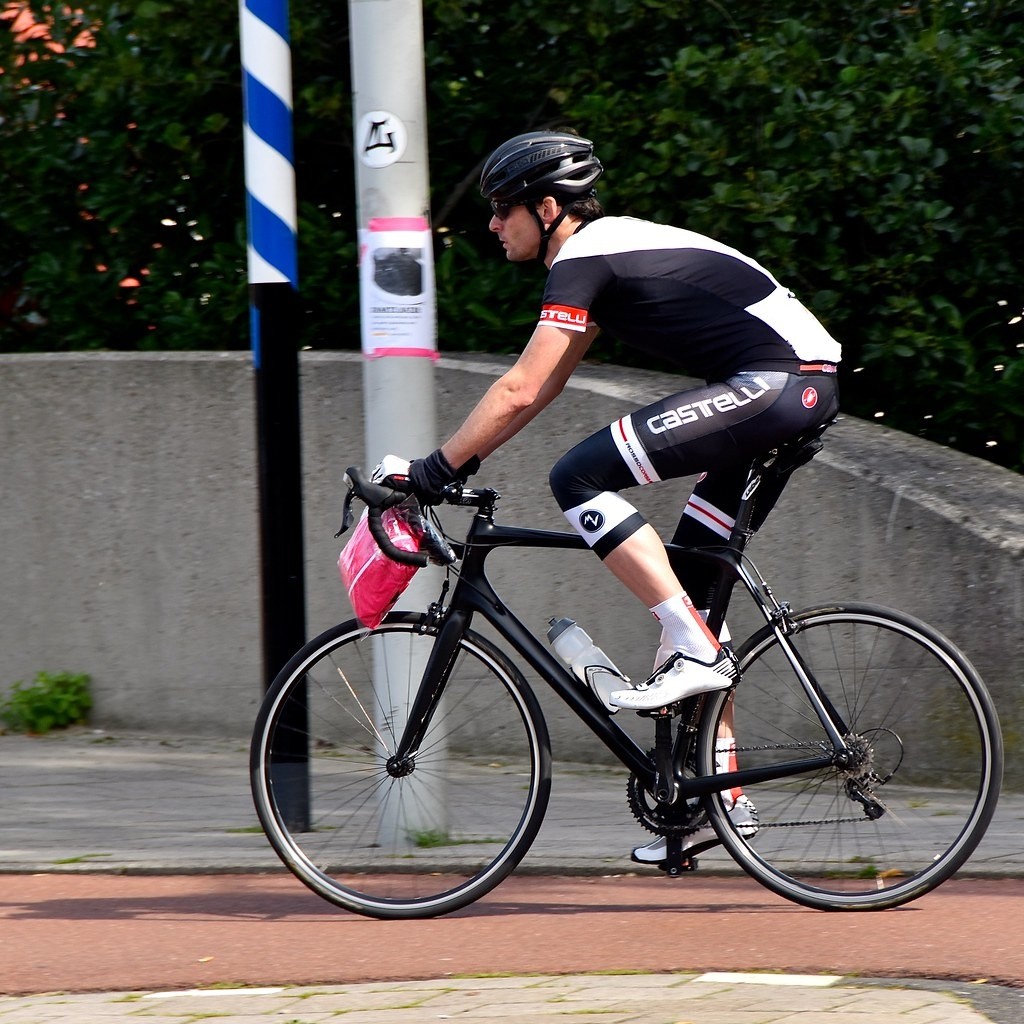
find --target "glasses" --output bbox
[490,197,542,221]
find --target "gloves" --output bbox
[367,449,481,506]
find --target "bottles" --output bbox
[546,617,635,713]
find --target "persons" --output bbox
[368,129,841,865]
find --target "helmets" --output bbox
[477,130,604,204]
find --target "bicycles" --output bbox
[247,424,1004,918]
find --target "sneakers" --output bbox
[608,645,742,710]
[630,794,760,864]
